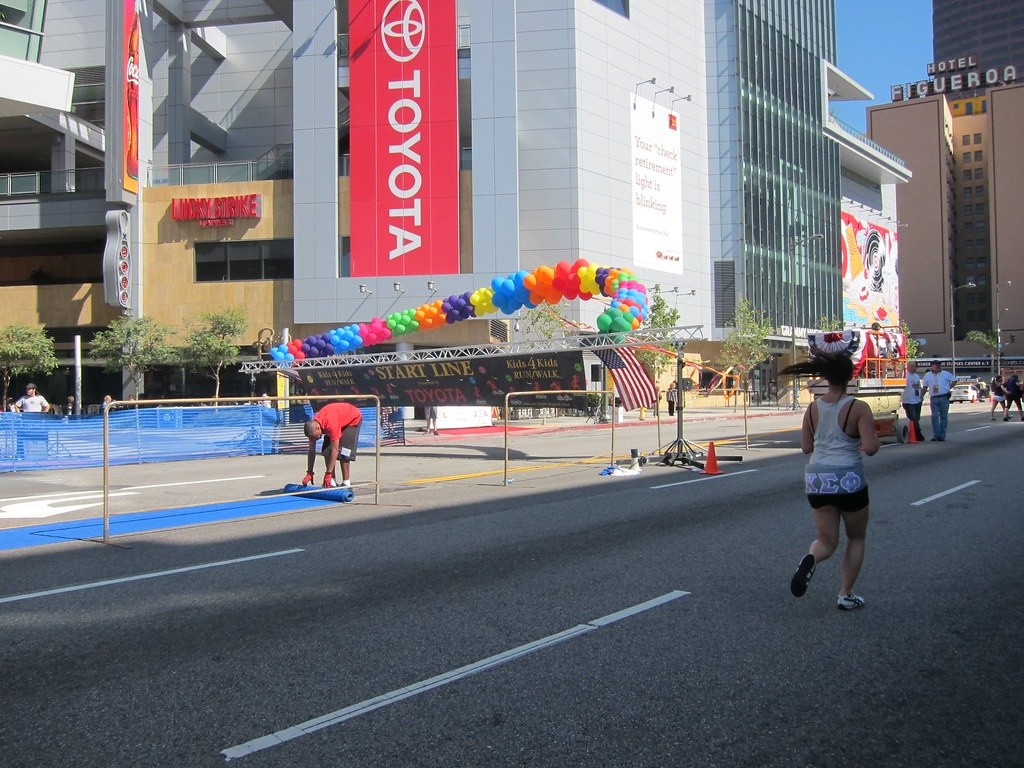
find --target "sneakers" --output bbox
[791,554,815,597]
[837,592,865,610]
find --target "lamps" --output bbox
[677,290,696,296]
[656,87,675,92]
[638,78,657,84]
[393,282,404,292]
[674,95,691,100]
[841,196,909,229]
[663,287,679,293]
[427,281,437,292]
[646,282,661,292]
[357,283,372,294]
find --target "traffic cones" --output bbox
[907,420,920,443]
[698,441,725,475]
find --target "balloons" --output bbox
[271,259,648,361]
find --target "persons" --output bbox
[302,403,362,488]
[423,406,439,435]
[977,379,986,399]
[871,323,887,358]
[7,403,16,411]
[901,361,924,440]
[103,395,136,412]
[922,361,957,441]
[790,354,880,610]
[14,383,50,417]
[666,383,677,416]
[991,368,1024,421]
[66,396,75,413]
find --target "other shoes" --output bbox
[1004,415,1013,421]
[423,430,431,436]
[341,483,352,489]
[932,435,944,441]
[331,483,338,487]
[434,431,438,435]
[916,435,924,441]
[991,417,995,420]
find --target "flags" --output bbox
[592,348,659,412]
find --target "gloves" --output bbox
[302,471,314,486]
[324,472,332,488]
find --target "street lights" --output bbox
[787,233,824,412]
[949,283,976,378]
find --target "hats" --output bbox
[931,360,941,365]
[25,383,36,389]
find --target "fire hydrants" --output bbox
[639,407,648,421]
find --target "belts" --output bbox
[932,395,948,398]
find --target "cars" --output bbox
[949,383,980,404]
